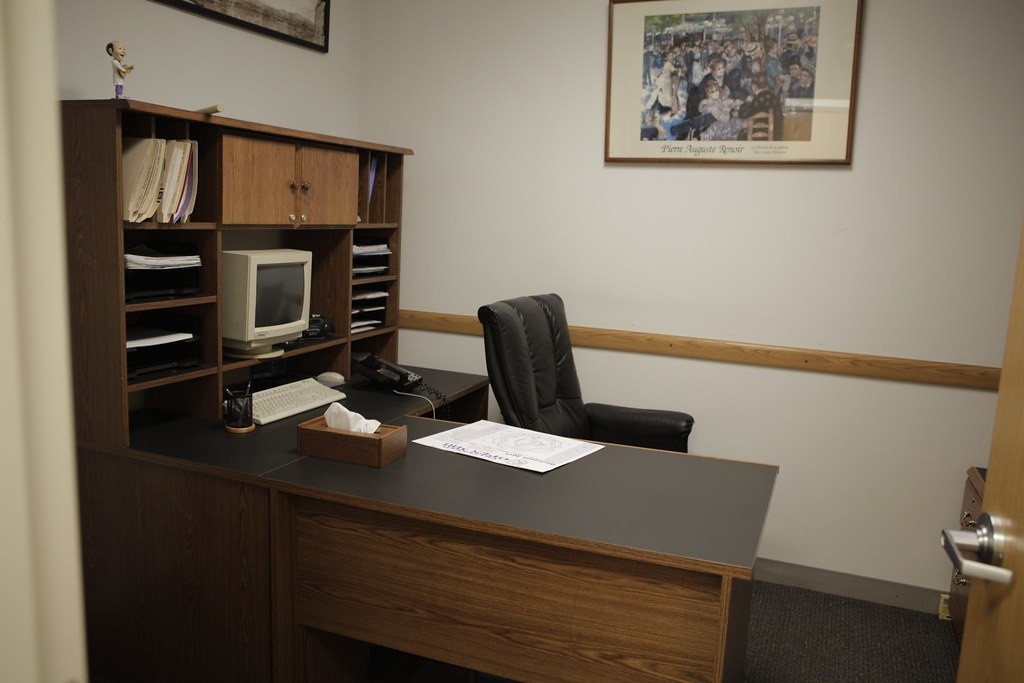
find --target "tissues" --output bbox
[297,403,408,470]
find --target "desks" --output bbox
[76,364,780,681]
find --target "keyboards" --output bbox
[251,377,346,425]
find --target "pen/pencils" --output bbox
[225,381,251,425]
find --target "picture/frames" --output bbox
[154,0,330,53]
[603,1,862,165]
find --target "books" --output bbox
[126,333,193,348]
[353,244,392,255]
[122,137,198,224]
[124,254,202,270]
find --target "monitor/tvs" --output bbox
[221,248,312,359]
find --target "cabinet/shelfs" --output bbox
[948,467,987,647]
[58,98,414,449]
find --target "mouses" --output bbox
[318,372,344,383]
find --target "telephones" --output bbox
[350,349,423,394]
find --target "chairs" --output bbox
[478,294,693,453]
